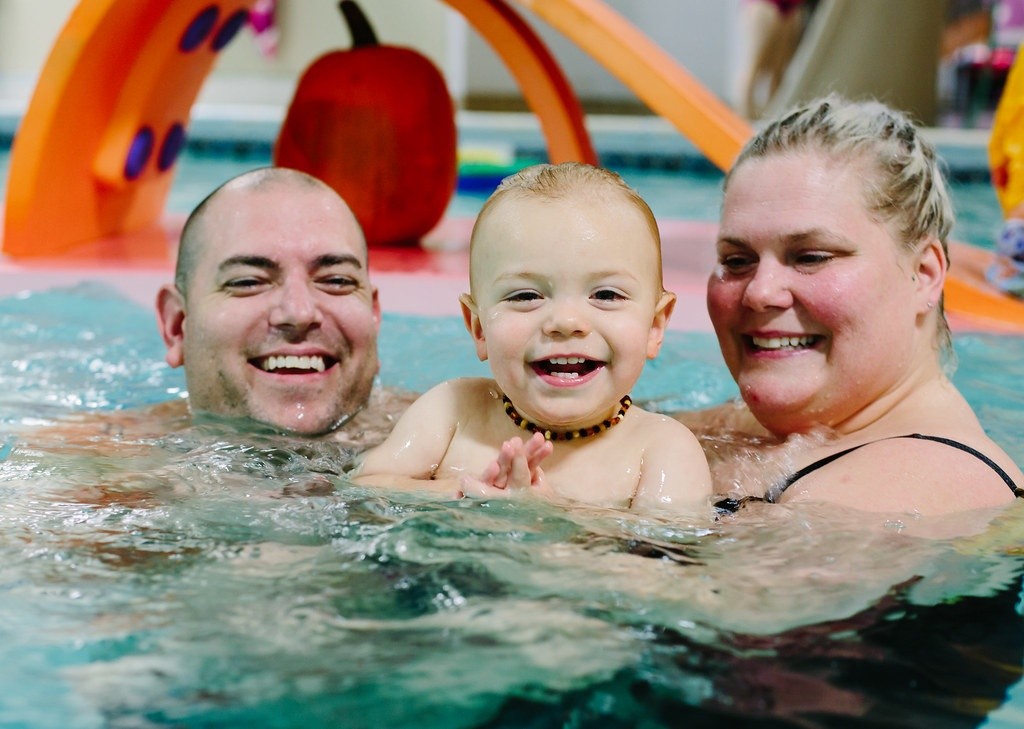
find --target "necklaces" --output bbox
[499,395,632,440]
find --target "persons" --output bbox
[10,168,421,461]
[700,95,1024,515]
[348,159,719,506]
